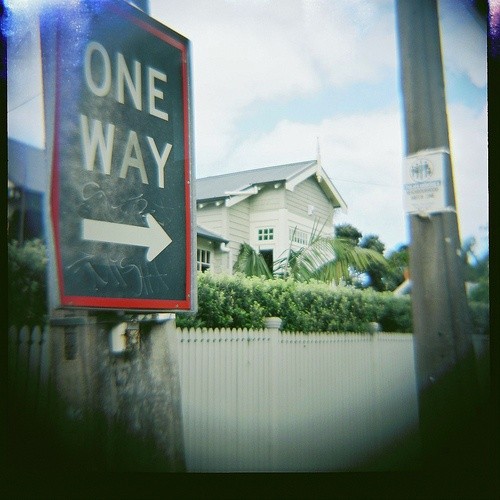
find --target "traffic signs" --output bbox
[38,1,199,315]
